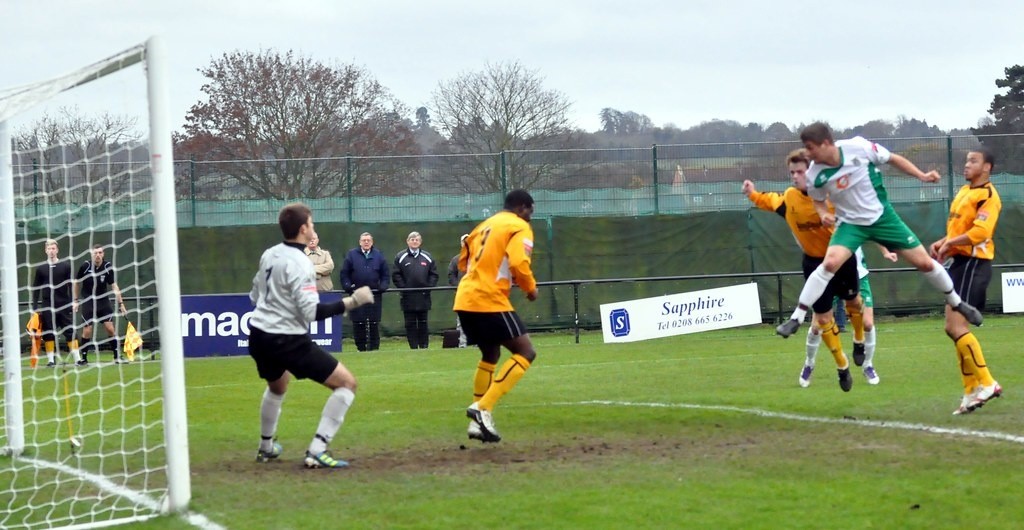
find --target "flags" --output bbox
[123,322,143,363]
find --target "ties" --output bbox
[414,253,417,256]
[364,251,369,259]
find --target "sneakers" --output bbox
[468,421,485,440]
[838,367,853,391]
[466,402,498,441]
[256,437,283,462]
[862,364,880,385]
[853,342,865,367]
[952,391,983,415]
[305,450,348,470]
[799,364,814,388]
[976,381,1002,402]
[776,318,800,339]
[955,302,984,327]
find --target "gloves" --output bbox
[352,286,376,307]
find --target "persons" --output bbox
[72,243,130,364]
[392,231,439,349]
[453,189,538,444]
[303,227,334,291]
[743,148,865,393]
[775,122,983,338]
[832,296,847,332]
[929,150,1002,412]
[797,240,898,387]
[339,232,390,351]
[248,203,374,468]
[449,234,478,347]
[31,239,86,366]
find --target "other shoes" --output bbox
[113,355,130,365]
[46,362,56,367]
[76,359,88,366]
[459,337,468,348]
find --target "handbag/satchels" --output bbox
[442,329,460,349]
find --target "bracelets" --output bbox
[119,302,124,304]
